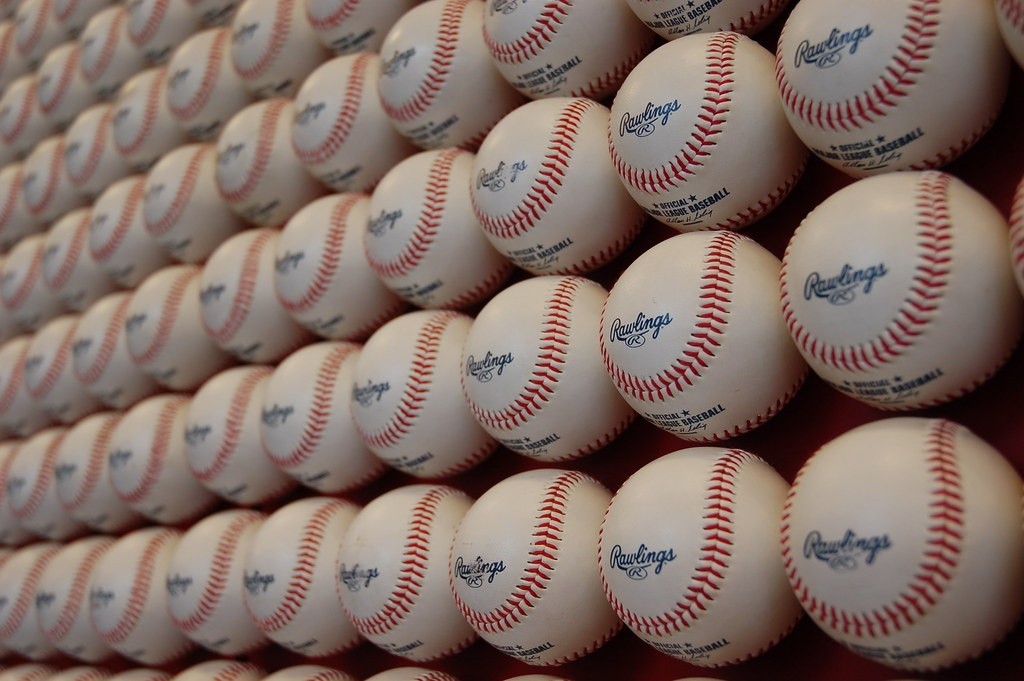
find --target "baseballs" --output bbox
[0,1,1024,681]
